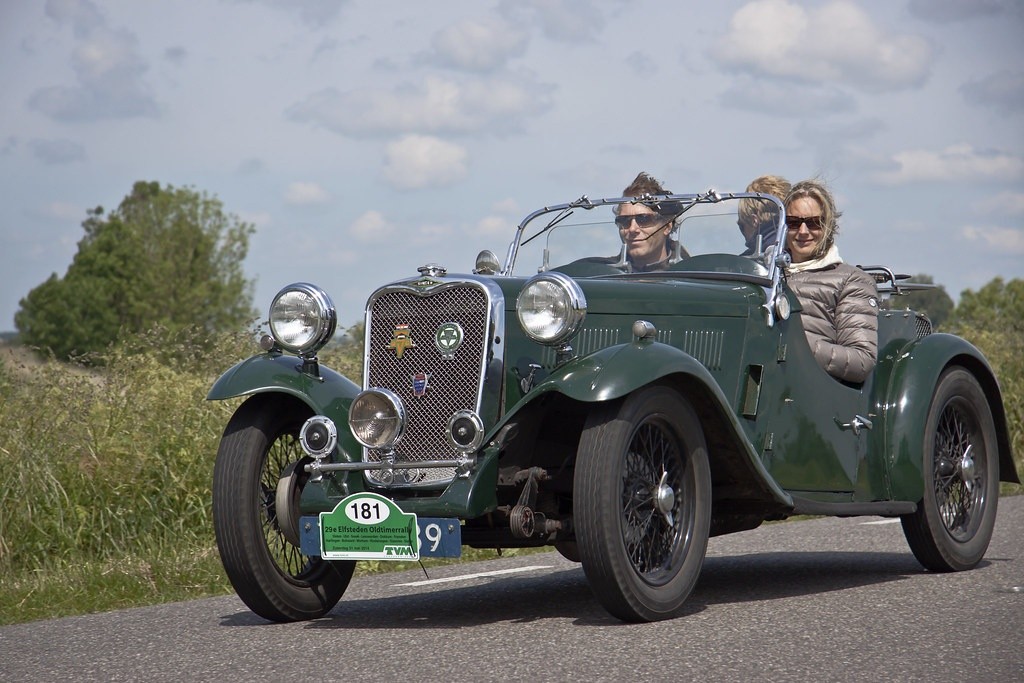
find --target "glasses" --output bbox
[615,213,663,229]
[786,216,826,230]
[738,221,748,233]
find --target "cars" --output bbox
[206,189,1024,624]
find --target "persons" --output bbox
[782,181,879,384]
[550,171,690,277]
[737,177,791,256]
